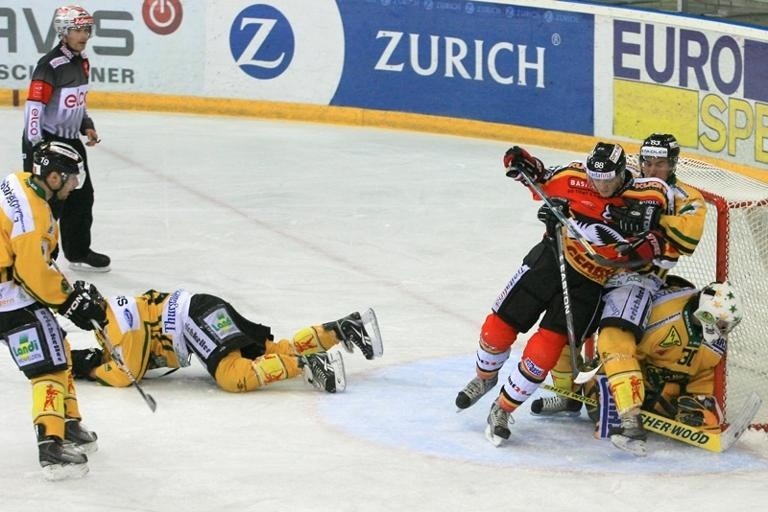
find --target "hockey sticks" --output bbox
[555,221,603,385]
[537,382,762,454]
[50,256,156,413]
[518,167,651,267]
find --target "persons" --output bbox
[0,139,99,480]
[58,288,384,393]
[454,141,676,446]
[530,133,705,457]
[584,274,743,440]
[22,5,111,273]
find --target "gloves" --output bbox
[503,146,544,186]
[617,231,663,272]
[674,395,725,436]
[57,290,109,331]
[70,347,103,381]
[609,197,664,233]
[537,199,569,241]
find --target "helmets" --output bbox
[53,5,93,44]
[638,133,680,186]
[32,141,86,190]
[71,281,106,311]
[585,142,626,192]
[689,280,744,348]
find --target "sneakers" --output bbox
[322,312,373,360]
[35,423,88,468]
[65,249,110,268]
[456,371,498,410]
[63,404,97,447]
[290,351,336,394]
[607,413,647,442]
[531,384,583,415]
[486,397,515,440]
